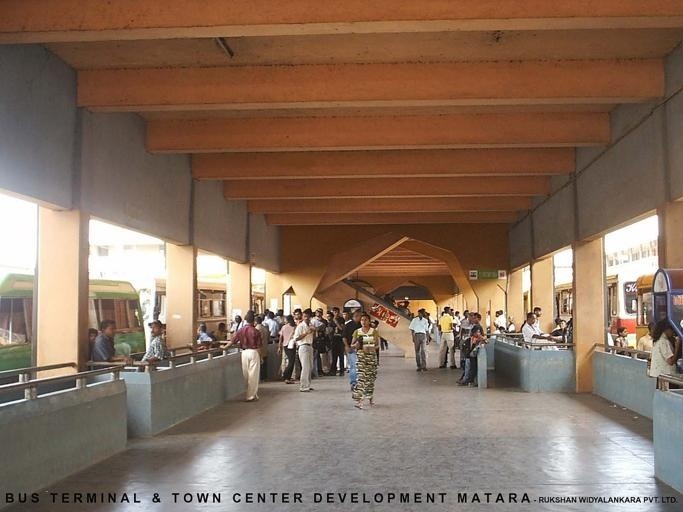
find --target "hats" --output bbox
[148,320,162,327]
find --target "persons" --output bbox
[606,322,616,354]
[88,327,97,353]
[136,320,167,372]
[615,327,628,354]
[196,310,378,408]
[398,300,574,385]
[94,319,132,367]
[380,336,389,352]
[649,322,681,389]
[637,322,657,360]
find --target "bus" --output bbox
[523,255,659,348]
[0,271,149,371]
[634,276,654,349]
[142,277,265,336]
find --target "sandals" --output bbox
[370,401,378,408]
[354,402,365,410]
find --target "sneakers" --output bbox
[300,387,314,392]
[423,368,428,372]
[416,368,422,371]
[456,380,466,385]
[245,395,260,401]
[450,365,457,369]
[282,367,349,384]
[439,365,447,368]
